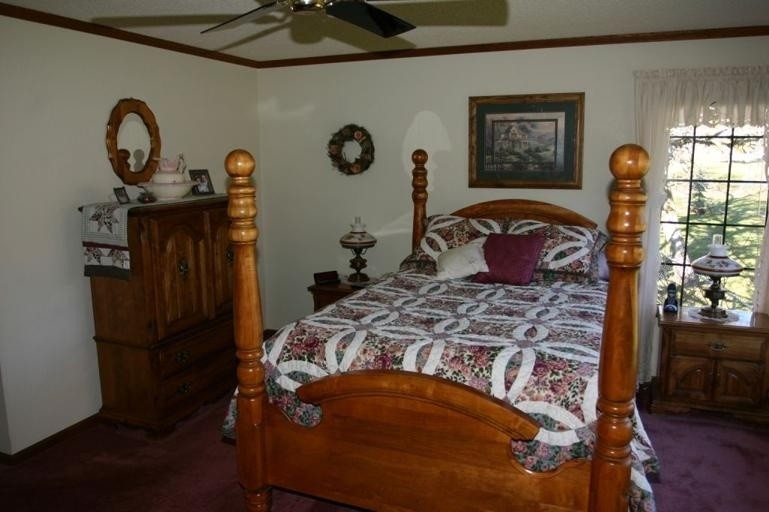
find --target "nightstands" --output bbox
[307,276,357,314]
[654,305,768,424]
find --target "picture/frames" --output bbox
[466,93,583,191]
[187,168,215,197]
[111,187,130,204]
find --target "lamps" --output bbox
[691,245,745,320]
[339,217,376,283]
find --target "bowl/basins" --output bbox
[137,181,200,201]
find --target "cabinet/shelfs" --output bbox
[78,195,239,432]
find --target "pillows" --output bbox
[435,242,489,281]
[506,220,601,276]
[472,231,542,284]
[403,215,505,269]
[533,234,608,283]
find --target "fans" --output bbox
[200,0,416,39]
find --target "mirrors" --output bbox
[104,99,160,186]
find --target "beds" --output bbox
[224,145,650,512]
